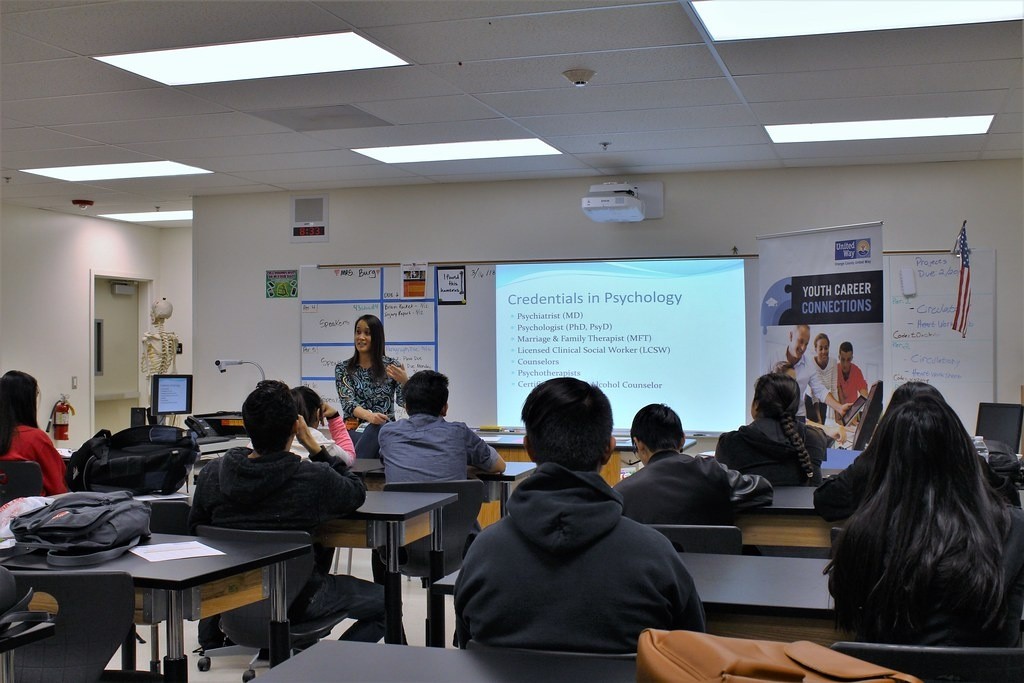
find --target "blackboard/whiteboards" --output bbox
[297,249,997,434]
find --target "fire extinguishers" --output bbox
[46,395,75,440]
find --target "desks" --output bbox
[349,458,537,525]
[435,549,850,650]
[247,639,642,683]
[474,433,696,490]
[335,489,458,649]
[734,484,836,549]
[6,531,310,683]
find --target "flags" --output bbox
[951,226,972,338]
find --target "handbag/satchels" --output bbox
[0,496,55,550]
[638,629,921,683]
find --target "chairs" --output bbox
[0,420,1024,683]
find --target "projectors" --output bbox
[582,196,646,222]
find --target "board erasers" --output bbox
[480,425,501,432]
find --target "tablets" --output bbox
[843,395,867,426]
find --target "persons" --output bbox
[188,380,385,643]
[612,404,774,524]
[247,386,356,467]
[714,372,828,486]
[0,370,69,497]
[813,381,1024,683]
[764,325,868,445]
[378,370,506,549]
[334,315,409,458]
[453,376,705,655]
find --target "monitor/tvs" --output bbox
[150,374,194,416]
[852,380,884,451]
[975,403,1024,454]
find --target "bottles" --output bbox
[973,436,989,463]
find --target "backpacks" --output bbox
[10,489,152,567]
[67,425,199,496]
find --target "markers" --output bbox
[694,434,707,436]
[504,429,523,433]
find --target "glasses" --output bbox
[632,442,641,461]
